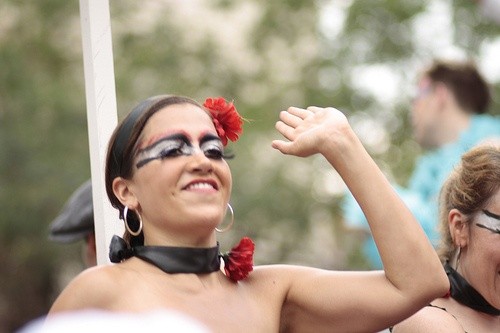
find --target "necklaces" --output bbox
[108,234,256,286]
[441,261,500,318]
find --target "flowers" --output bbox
[202,97,243,145]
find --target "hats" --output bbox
[48,178,95,241]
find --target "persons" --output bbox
[47,181,100,270]
[389,137,500,333]
[41,92,450,333]
[336,56,500,271]
